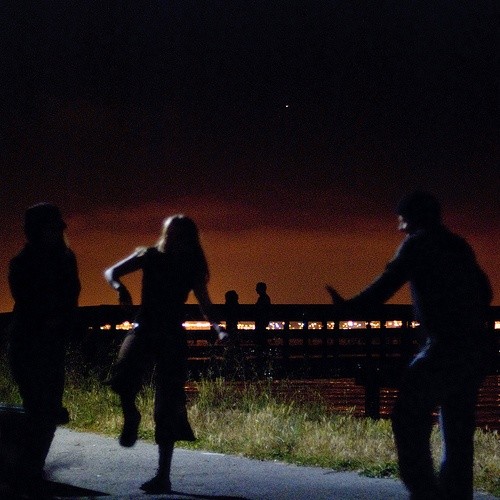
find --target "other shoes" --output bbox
[119,411,141,447]
[139,474,171,495]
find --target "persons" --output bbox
[223,289,241,346]
[102,214,228,494]
[254,280,272,350]
[9,202,81,474]
[325,193,494,499]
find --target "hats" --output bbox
[22,201,68,238]
[394,191,440,221]
